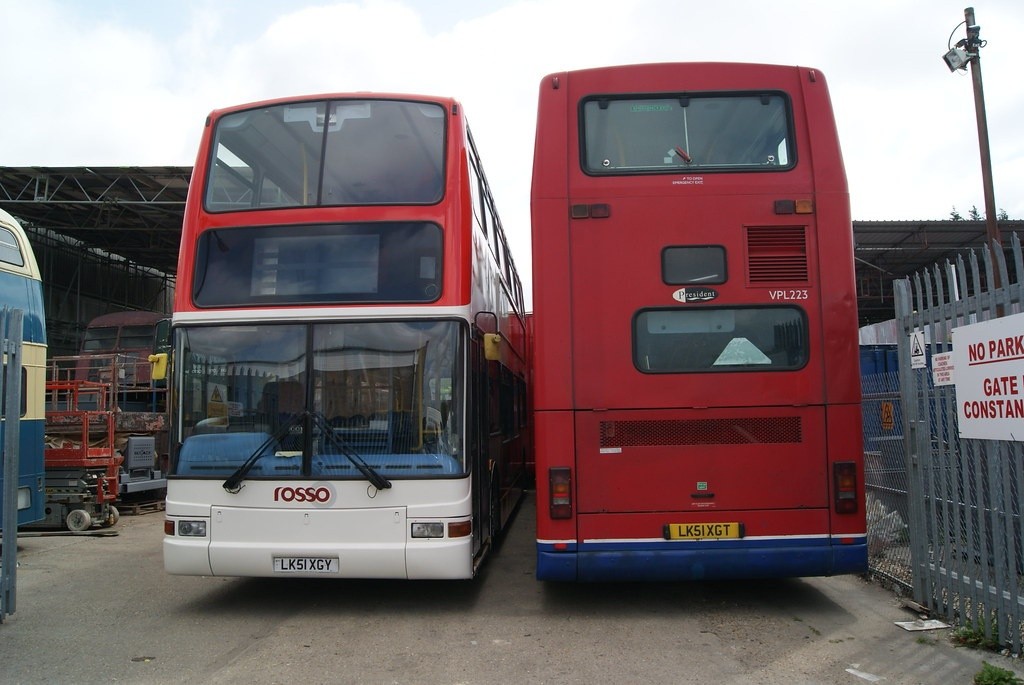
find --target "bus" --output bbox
[529,62,869,581]
[148,91,529,581]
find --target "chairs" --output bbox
[220,407,412,452]
